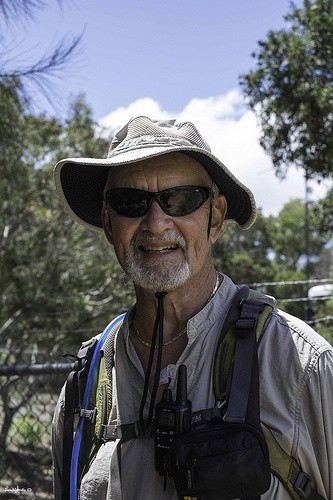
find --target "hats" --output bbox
[53,117,257,230]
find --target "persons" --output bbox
[43,113,332,499]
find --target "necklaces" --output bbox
[131,267,222,349]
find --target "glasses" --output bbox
[104,185,212,217]
